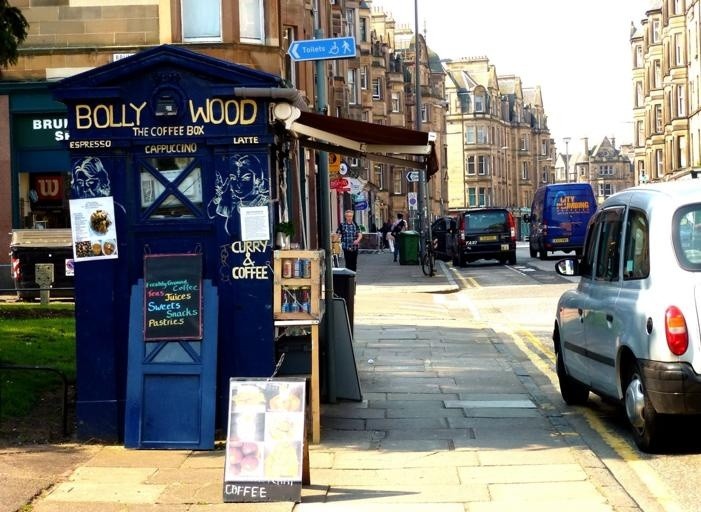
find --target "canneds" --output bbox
[282,259,292,277]
[292,261,302,278]
[290,287,300,312]
[282,287,291,312]
[300,286,310,312]
[302,260,311,278]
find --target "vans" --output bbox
[523,184,598,261]
[431,209,517,265]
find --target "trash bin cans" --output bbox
[398,230,420,265]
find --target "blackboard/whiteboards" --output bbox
[144,253,203,342]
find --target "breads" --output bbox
[91,211,108,233]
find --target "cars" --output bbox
[552,180,700,453]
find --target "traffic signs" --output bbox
[288,36,359,63]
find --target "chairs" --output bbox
[33,214,48,229]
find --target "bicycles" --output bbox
[421,239,435,276]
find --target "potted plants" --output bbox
[276,221,296,250]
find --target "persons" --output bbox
[334,208,362,272]
[376,213,409,262]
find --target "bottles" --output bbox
[282,286,310,313]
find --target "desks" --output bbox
[273,299,326,444]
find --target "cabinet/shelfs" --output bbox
[274,250,325,321]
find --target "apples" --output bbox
[229,442,259,474]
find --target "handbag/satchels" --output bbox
[394,220,405,232]
